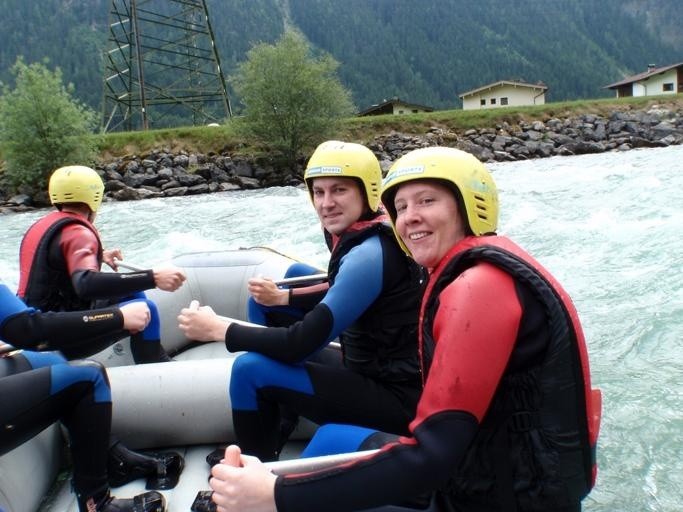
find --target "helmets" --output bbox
[304,139,499,259]
[48,165,105,213]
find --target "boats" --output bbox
[0,245,344,512]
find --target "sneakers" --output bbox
[79,488,165,511]
[206,448,224,466]
[109,441,178,489]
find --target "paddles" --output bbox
[222,388,601,481]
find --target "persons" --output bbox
[206,143,604,512]
[1,273,186,512]
[15,162,188,364]
[174,137,427,468]
